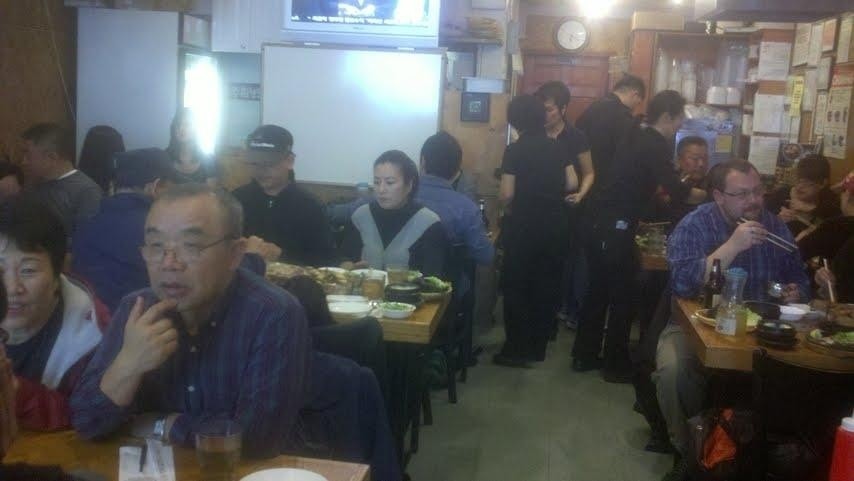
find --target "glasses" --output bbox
[792,176,819,187]
[717,185,764,201]
[137,235,241,266]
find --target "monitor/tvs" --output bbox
[279,1,441,51]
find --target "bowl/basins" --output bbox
[327,302,373,318]
[379,303,415,318]
[776,305,804,321]
[747,302,779,317]
[706,86,741,104]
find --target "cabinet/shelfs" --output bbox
[439,35,503,93]
[628,28,795,184]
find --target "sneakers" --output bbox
[572,352,641,384]
[492,348,544,369]
[555,305,611,332]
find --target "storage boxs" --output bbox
[630,11,685,33]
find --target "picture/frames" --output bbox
[460,93,490,124]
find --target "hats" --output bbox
[240,124,293,167]
[830,170,854,193]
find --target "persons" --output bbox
[498,77,854,461]
[1,78,497,480]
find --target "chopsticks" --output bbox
[735,215,799,253]
[821,258,837,305]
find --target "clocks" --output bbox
[553,15,589,57]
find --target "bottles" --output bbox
[478,200,488,226]
[705,258,724,308]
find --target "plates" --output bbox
[239,467,328,481]
[759,322,802,347]
[351,267,387,285]
[407,278,452,299]
[411,270,424,277]
[696,310,761,332]
[319,266,349,275]
[326,292,369,302]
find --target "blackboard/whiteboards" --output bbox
[259,43,446,186]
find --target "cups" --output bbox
[362,274,384,300]
[388,264,411,292]
[654,46,750,102]
[193,422,242,477]
[718,268,747,341]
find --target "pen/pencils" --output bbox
[138,444,146,472]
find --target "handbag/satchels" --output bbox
[668,398,766,481]
